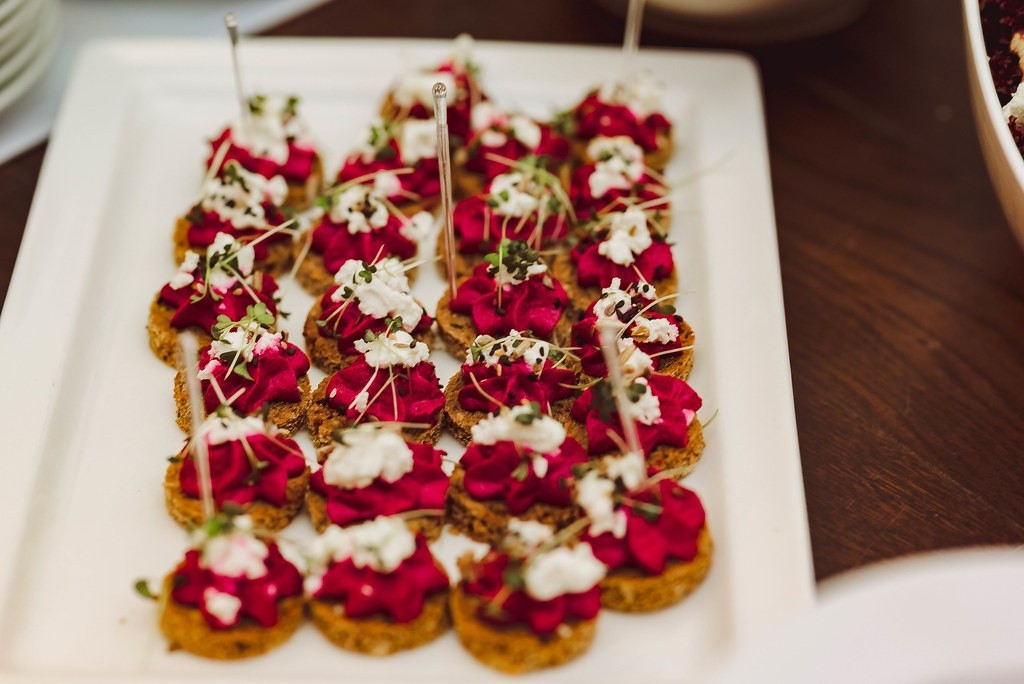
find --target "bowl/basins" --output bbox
[962,0,1024,253]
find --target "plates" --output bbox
[810,544,1024,684]
[0,34,817,683]
[0,0,325,163]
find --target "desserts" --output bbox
[146,32,716,674]
